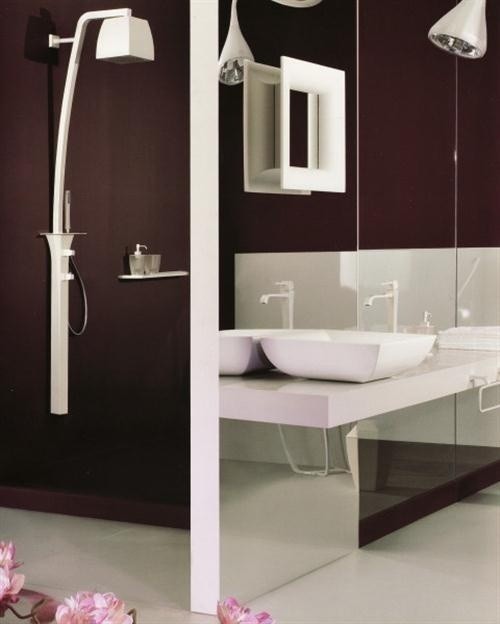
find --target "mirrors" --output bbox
[219,0,500,331]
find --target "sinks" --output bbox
[258,330,437,384]
[217,328,271,376]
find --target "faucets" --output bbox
[363,279,401,333]
[259,281,296,328]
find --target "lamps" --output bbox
[429,0,488,60]
[219,0,255,86]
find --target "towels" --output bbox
[437,327,499,349]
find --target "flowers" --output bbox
[0,540,140,623]
[216,597,273,624]
